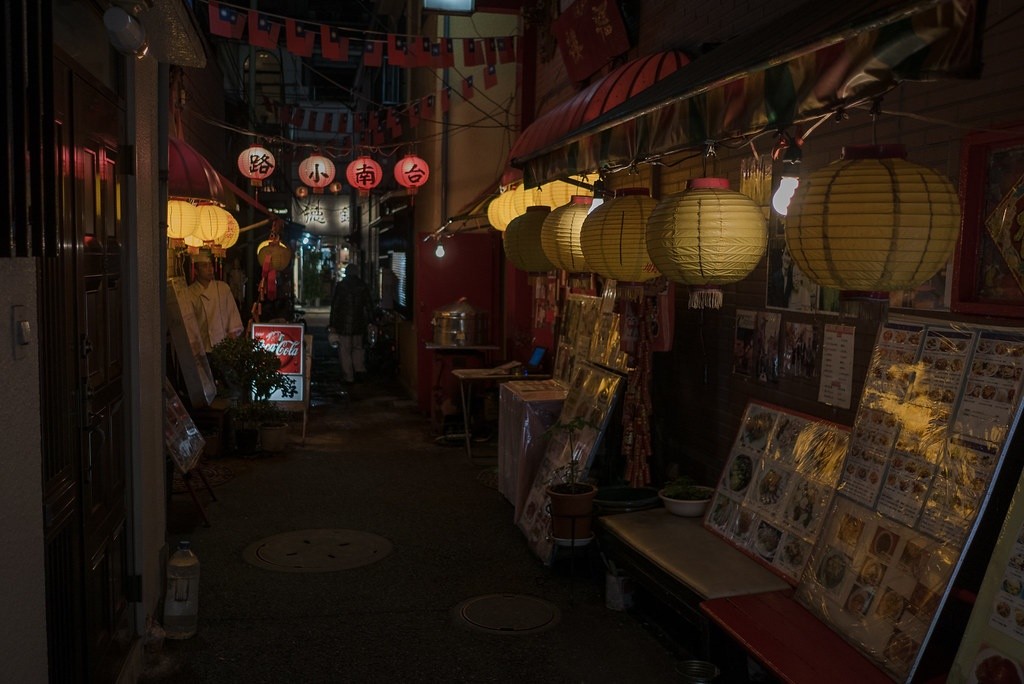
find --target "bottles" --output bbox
[367,322,378,344]
[162,541,200,641]
[327,327,340,349]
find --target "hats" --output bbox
[194,249,212,262]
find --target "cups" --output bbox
[672,660,722,684]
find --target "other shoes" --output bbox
[355,372,363,381]
[339,377,353,385]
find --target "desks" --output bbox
[425,341,500,452]
[449,366,550,457]
[603,510,794,600]
[699,593,900,684]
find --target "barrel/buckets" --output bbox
[595,484,662,516]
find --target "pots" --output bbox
[430,301,490,347]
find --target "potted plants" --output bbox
[536,406,601,542]
[258,404,292,458]
[659,475,715,517]
[208,335,299,459]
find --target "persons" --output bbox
[329,264,373,393]
[188,252,243,355]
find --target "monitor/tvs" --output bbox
[528,345,547,368]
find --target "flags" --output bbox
[208,0,516,158]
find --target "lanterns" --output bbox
[299,154,335,194]
[237,144,276,186]
[787,144,960,324]
[503,177,766,312]
[167,200,240,279]
[487,174,598,231]
[393,153,429,195]
[346,155,384,196]
[257,239,290,273]
